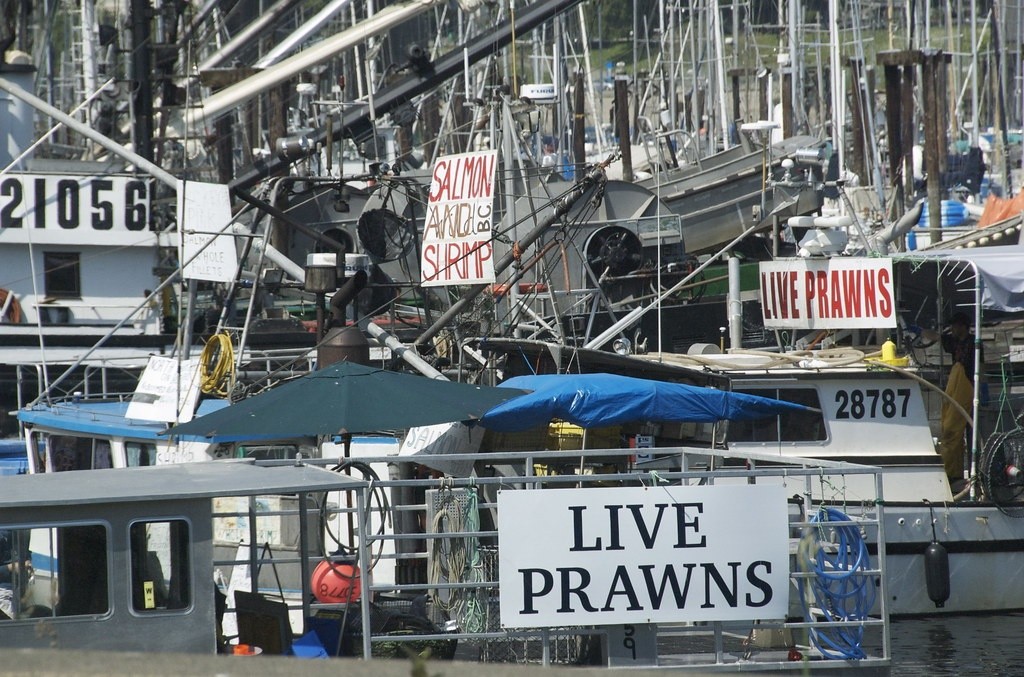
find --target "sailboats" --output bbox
[2,0,1022,677]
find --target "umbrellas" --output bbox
[157,358,536,555]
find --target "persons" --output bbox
[144,283,224,322]
[0,530,35,620]
[540,135,574,185]
[907,313,985,481]
